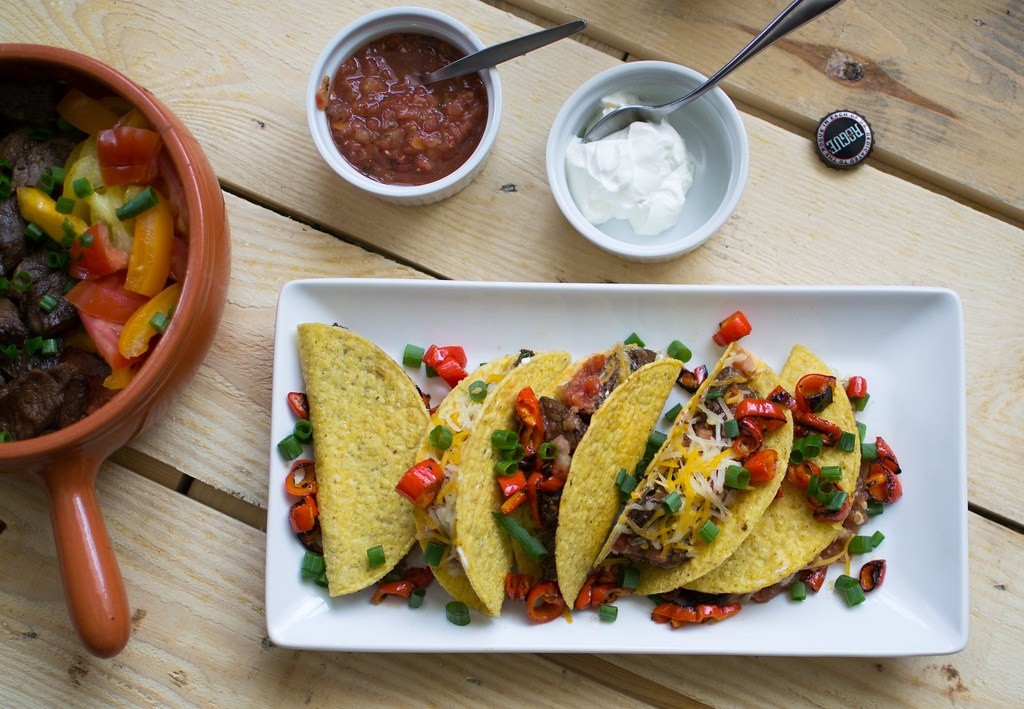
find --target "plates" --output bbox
[266,276,969,663]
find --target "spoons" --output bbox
[584,3,836,138]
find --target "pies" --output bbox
[297,319,865,599]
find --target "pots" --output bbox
[0,41,231,662]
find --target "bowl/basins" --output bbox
[304,10,500,208]
[547,60,747,262]
